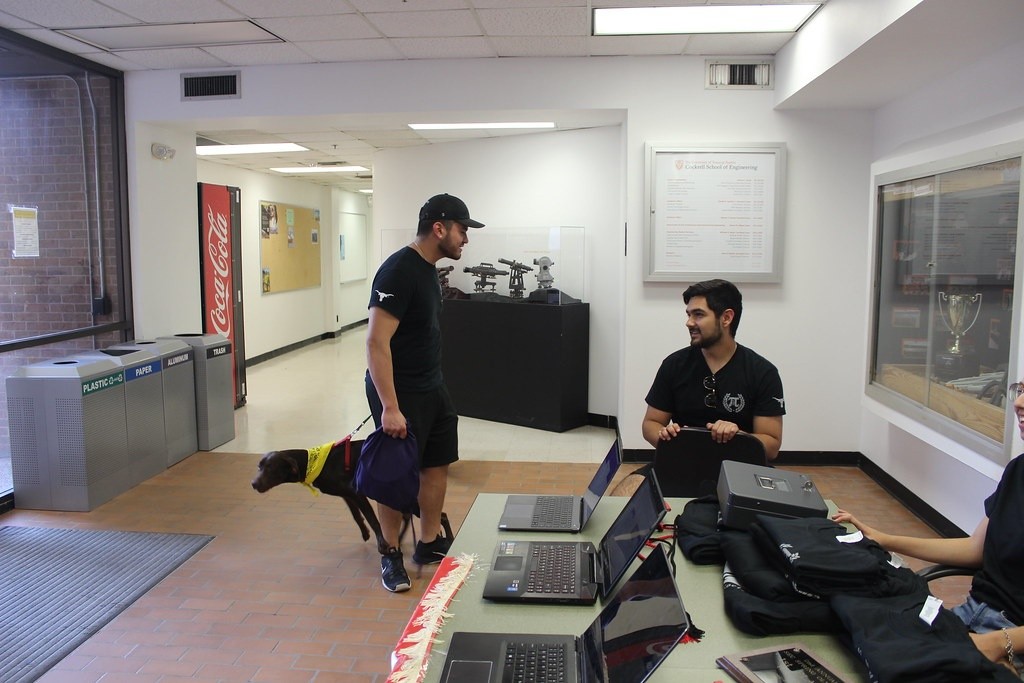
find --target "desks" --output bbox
[390,491,861,683]
[439,300,591,432]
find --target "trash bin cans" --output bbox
[108,337,200,468]
[155,334,236,452]
[68,347,169,489]
[5,357,130,513]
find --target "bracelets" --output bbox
[1002,627,1014,664]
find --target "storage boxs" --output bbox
[376,225,586,307]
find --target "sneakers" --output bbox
[381,547,412,592]
[412,533,454,564]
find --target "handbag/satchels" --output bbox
[354,421,420,518]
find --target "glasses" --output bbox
[1008,383,1024,402]
[703,375,718,408]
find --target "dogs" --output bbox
[250,440,456,544]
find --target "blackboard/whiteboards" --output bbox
[338,211,368,284]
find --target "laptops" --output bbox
[440,436,690,683]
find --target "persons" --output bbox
[364,193,485,592]
[832,378,1024,683]
[609,279,787,497]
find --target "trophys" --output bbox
[936,290,983,381]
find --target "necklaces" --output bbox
[413,241,426,260]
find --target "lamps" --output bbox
[151,142,176,161]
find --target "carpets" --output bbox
[0,524,216,683]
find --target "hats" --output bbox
[419,193,486,228]
